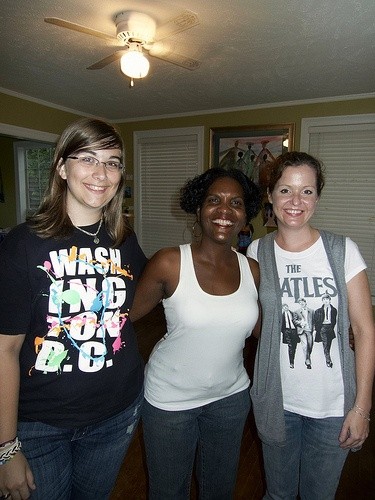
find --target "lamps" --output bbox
[282,135,289,147]
[115,11,157,87]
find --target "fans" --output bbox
[44,9,202,72]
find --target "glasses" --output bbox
[65,155,126,173]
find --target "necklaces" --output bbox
[74,213,103,245]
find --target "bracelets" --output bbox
[353,404,371,416]
[0,436,22,465]
[350,408,370,422]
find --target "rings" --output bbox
[3,493,11,499]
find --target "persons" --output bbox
[246,151,374,500]
[128,168,355,500]
[0,118,148,500]
